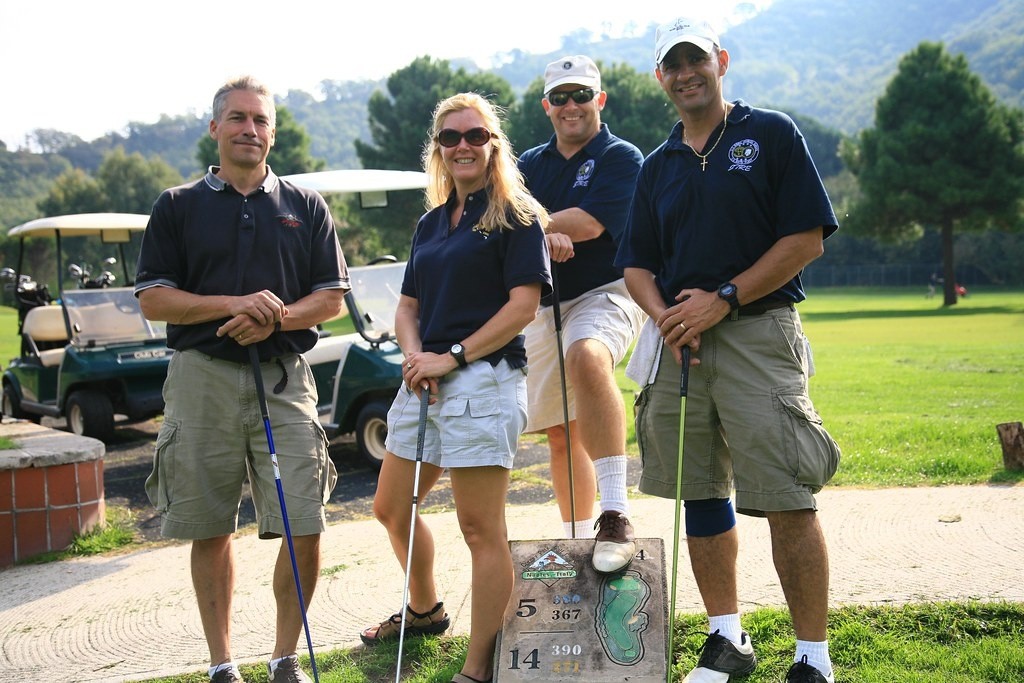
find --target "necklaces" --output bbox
[683,102,727,171]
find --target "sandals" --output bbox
[360,600,449,644]
[451,672,495,683]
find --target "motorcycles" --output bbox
[0,257,116,357]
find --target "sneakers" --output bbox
[267,650,314,683]
[209,658,246,683]
[591,511,636,573]
[679,629,758,683]
[785,653,835,683]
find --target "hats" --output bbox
[543,55,602,97]
[655,17,721,68]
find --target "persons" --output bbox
[613,11,840,683]
[359,88,554,683]
[515,55,645,576]
[925,273,938,297]
[134,75,351,683]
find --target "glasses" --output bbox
[437,126,500,148]
[549,88,601,106]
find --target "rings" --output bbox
[407,363,411,369]
[239,335,244,339]
[679,323,686,330]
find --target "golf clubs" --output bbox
[551,257,575,538]
[249,343,319,683]
[667,345,691,683]
[67,256,118,289]
[0,266,48,293]
[396,387,430,683]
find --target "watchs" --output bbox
[718,281,739,309]
[449,343,467,368]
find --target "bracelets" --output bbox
[272,321,281,333]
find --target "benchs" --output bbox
[20,304,145,368]
[303,331,399,407]
